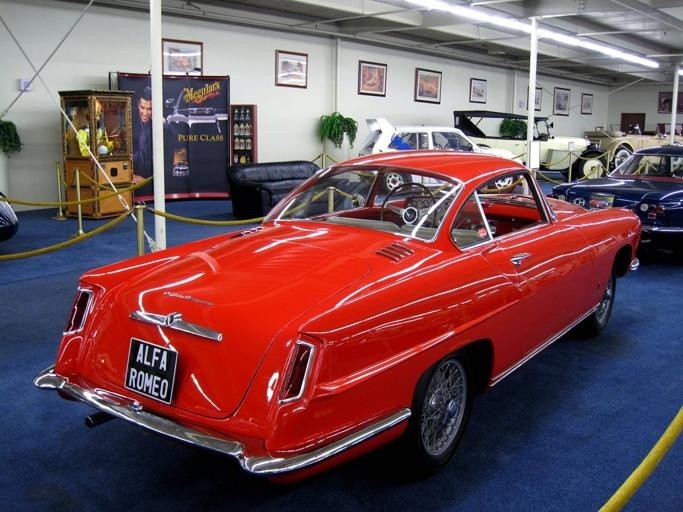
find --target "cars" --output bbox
[163,88,228,138]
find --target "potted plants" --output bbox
[320,112,358,168]
[0,119,24,199]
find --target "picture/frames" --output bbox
[162,38,203,76]
[275,49,308,88]
[358,60,388,96]
[553,87,570,115]
[580,93,593,114]
[415,68,442,105]
[658,91,683,114]
[469,78,487,103]
[527,86,542,111]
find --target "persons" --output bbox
[131,87,177,184]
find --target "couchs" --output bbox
[226,161,320,219]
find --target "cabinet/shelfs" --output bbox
[231,104,258,166]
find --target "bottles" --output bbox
[233,107,252,164]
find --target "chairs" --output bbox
[398,223,479,247]
[326,217,398,232]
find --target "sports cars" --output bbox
[34,152,643,483]
